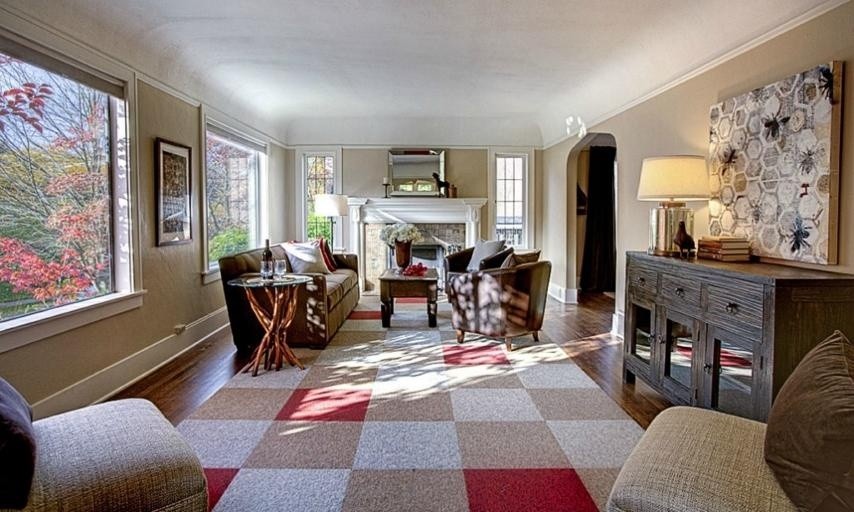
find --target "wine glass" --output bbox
[274,259,287,281]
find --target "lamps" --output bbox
[637,155,711,257]
[313,194,349,252]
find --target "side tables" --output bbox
[227,274,313,376]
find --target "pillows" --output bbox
[499,250,540,269]
[764,329,853,512]
[280,238,337,273]
[0,375,36,510]
[466,239,507,270]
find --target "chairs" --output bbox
[28,398,207,512]
[607,406,854,511]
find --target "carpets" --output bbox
[180,294,644,512]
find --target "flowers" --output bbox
[378,223,424,247]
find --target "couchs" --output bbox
[444,245,512,303]
[218,246,359,350]
[450,260,552,351]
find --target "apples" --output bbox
[404,263,427,277]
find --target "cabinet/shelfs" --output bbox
[622,249,702,407]
[703,265,854,422]
[385,150,446,198]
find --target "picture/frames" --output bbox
[153,136,193,248]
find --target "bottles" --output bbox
[261,238,274,280]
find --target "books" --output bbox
[697,251,750,262]
[702,236,746,241]
[698,247,749,255]
[698,239,750,249]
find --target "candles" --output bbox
[384,177,389,184]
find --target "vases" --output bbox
[394,238,413,274]
